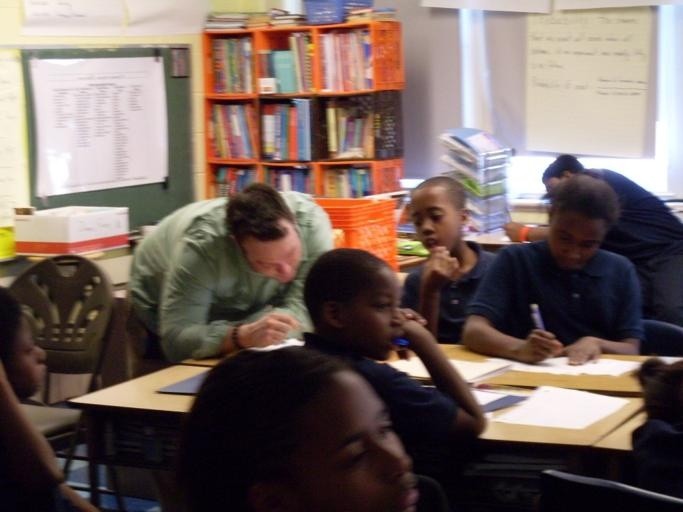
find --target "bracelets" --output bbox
[231,321,246,350]
[518,225,530,242]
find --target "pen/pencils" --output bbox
[392,339,409,346]
[531,304,545,332]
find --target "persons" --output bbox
[123,181,335,364]
[457,174,645,366]
[395,175,498,348]
[297,246,484,511]
[500,152,682,329]
[0,284,103,512]
[152,345,420,512]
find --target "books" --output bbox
[201,13,375,202]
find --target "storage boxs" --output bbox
[15,206,130,258]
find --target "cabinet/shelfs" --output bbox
[199,21,405,202]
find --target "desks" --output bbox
[394,208,553,269]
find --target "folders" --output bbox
[441,124,514,230]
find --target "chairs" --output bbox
[0,254,129,512]
[639,319,683,357]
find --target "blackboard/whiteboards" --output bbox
[0,45,196,230]
